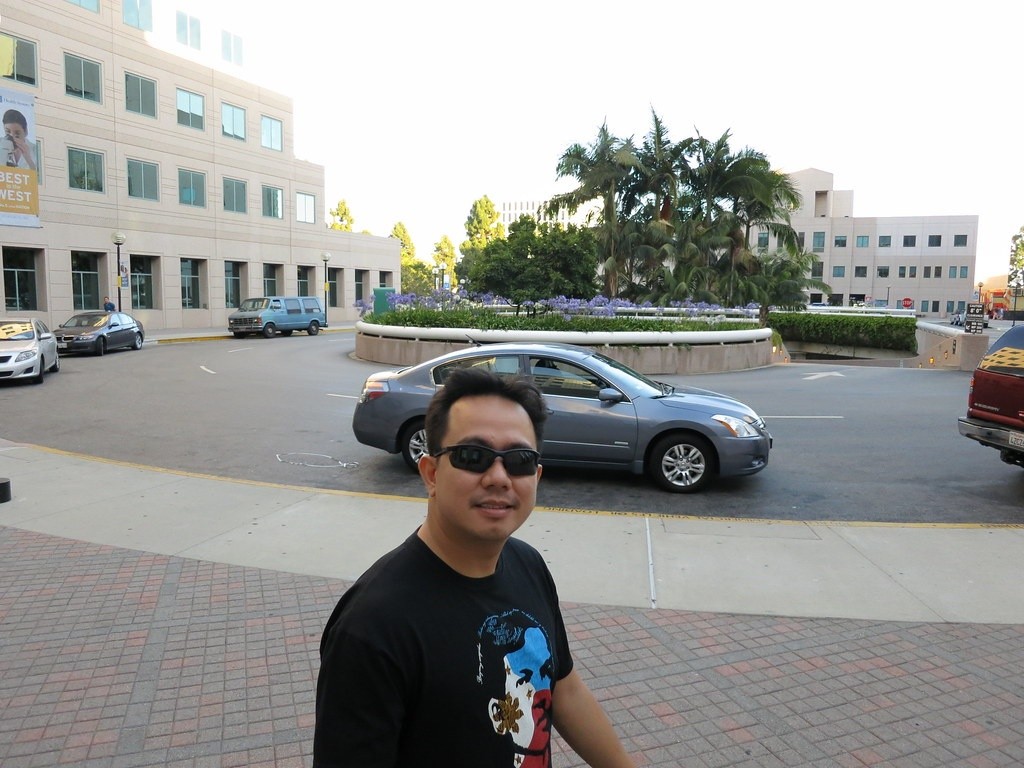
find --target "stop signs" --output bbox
[903,298,912,307]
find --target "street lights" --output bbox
[431,262,447,311]
[320,251,331,327]
[111,231,127,312]
[886,285,891,304]
[977,282,983,303]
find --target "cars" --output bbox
[0,316,61,385]
[352,341,773,494]
[54,311,145,356]
[957,322,1024,469]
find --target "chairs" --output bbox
[532,359,560,386]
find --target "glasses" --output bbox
[433,444,540,476]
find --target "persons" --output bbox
[0,109,36,171]
[103,296,115,312]
[313,367,641,768]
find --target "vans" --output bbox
[228,296,326,339]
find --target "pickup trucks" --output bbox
[950,309,989,328]
